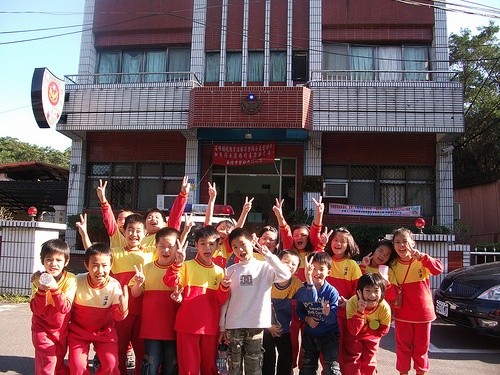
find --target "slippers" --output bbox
[93,355,99,371]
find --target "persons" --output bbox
[388,227,444,375]
[28,239,78,375]
[263,248,318,375]
[162,225,236,375]
[96,175,394,375]
[76,212,197,375]
[67,243,130,375]
[218,227,292,375]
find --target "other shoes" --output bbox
[126,350,135,367]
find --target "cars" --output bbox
[434,260,500,340]
[167,204,238,247]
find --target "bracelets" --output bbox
[307,283,316,287]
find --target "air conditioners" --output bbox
[157,195,177,210]
[323,183,348,198]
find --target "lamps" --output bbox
[416,218,425,233]
[28,207,37,221]
